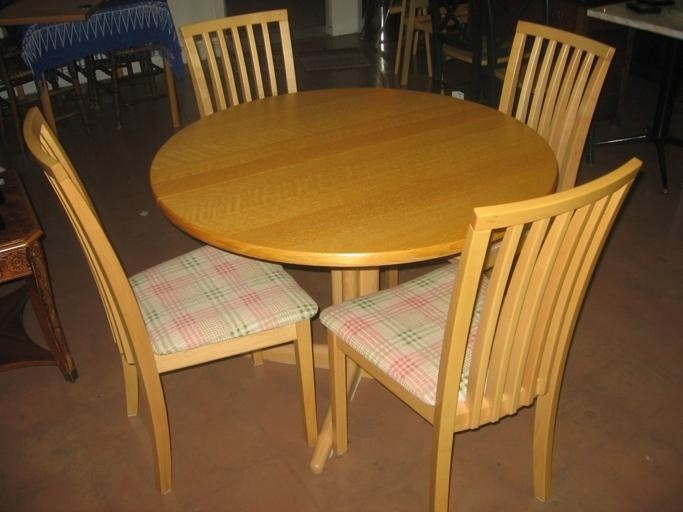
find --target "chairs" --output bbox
[176,9,301,121]
[431,18,617,274]
[22,106,321,496]
[2,166,80,386]
[2,0,182,156]
[317,157,642,511]
[366,1,552,104]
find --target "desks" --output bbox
[586,0,683,194]
[149,84,560,478]
